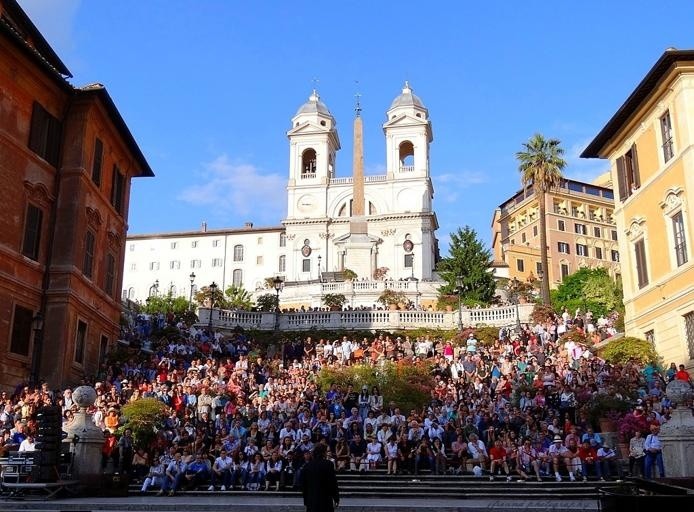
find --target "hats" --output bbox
[552,435,563,444]
[601,442,611,448]
[107,407,118,414]
[121,379,128,384]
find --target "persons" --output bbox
[298,441,341,512]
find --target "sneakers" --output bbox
[489,475,606,483]
[139,485,280,497]
